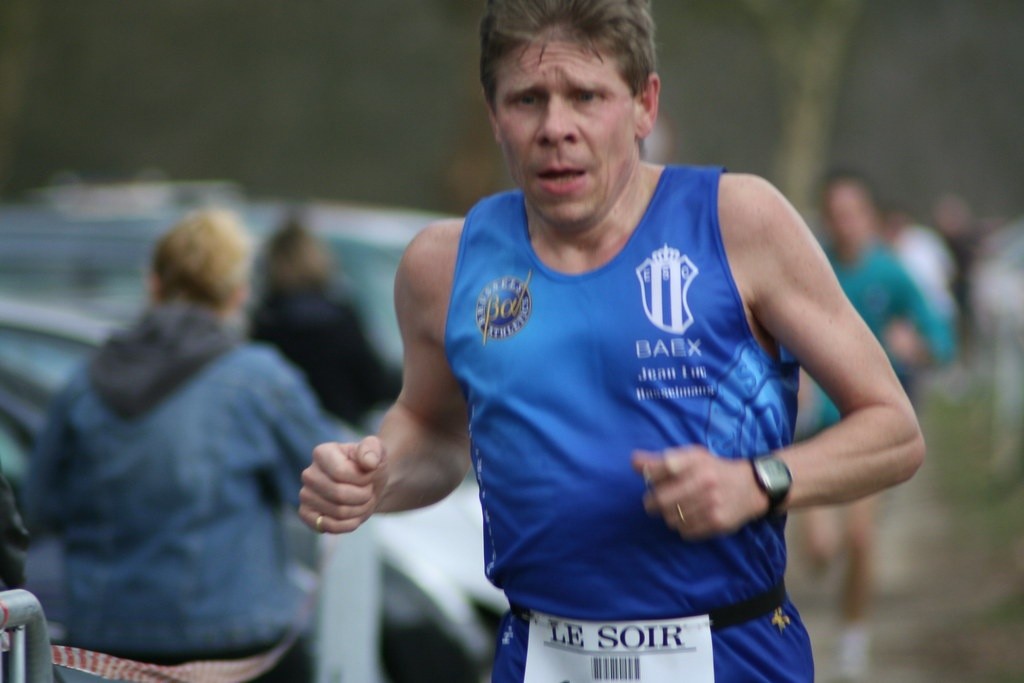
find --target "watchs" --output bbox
[750,454,793,522]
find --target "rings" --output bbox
[316,514,324,534]
[677,504,687,523]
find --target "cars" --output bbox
[0,184,505,683]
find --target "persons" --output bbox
[793,165,1024,683]
[21,208,353,682]
[253,223,407,428]
[298,0,923,683]
